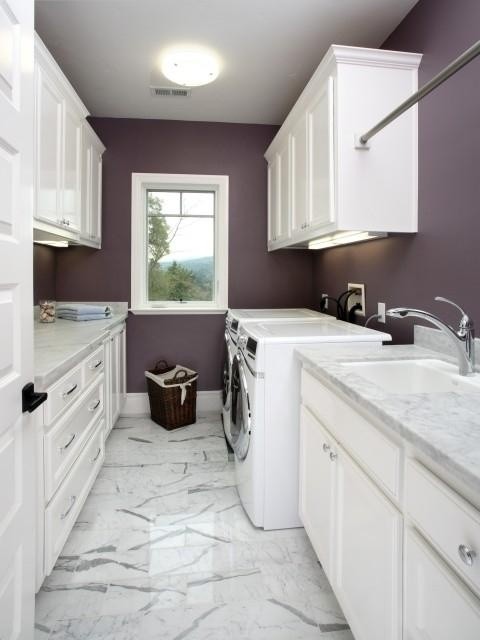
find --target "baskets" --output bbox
[145,360,198,430]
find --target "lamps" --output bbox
[157,42,226,89]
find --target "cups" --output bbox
[38,299,57,323]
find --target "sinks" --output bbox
[340,358,480,397]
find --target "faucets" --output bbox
[383,295,476,375]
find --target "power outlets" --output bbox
[376,303,389,322]
[320,293,330,310]
[347,286,364,314]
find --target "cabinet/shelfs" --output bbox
[80,122,102,253]
[106,320,129,434]
[36,55,80,234]
[401,441,480,640]
[265,131,295,254]
[44,337,108,577]
[289,61,337,243]
[300,363,401,640]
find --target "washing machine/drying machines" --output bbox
[219,306,339,462]
[222,320,392,531]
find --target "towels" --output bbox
[40,300,115,322]
